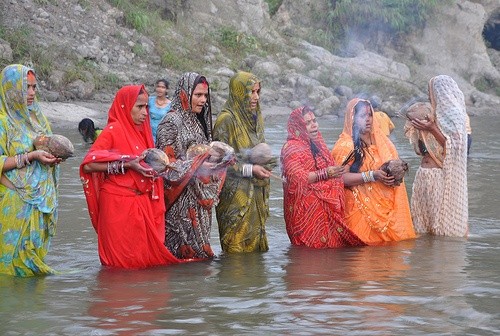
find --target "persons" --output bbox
[212,72,277,253]
[0,64,66,278]
[331,97,403,243]
[146,71,239,261]
[78,118,104,143]
[360,93,399,142]
[404,75,471,237]
[79,84,178,269]
[280,106,368,249]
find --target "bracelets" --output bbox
[315,168,328,181]
[107,161,126,176]
[243,163,253,178]
[15,152,31,168]
[362,170,378,183]
